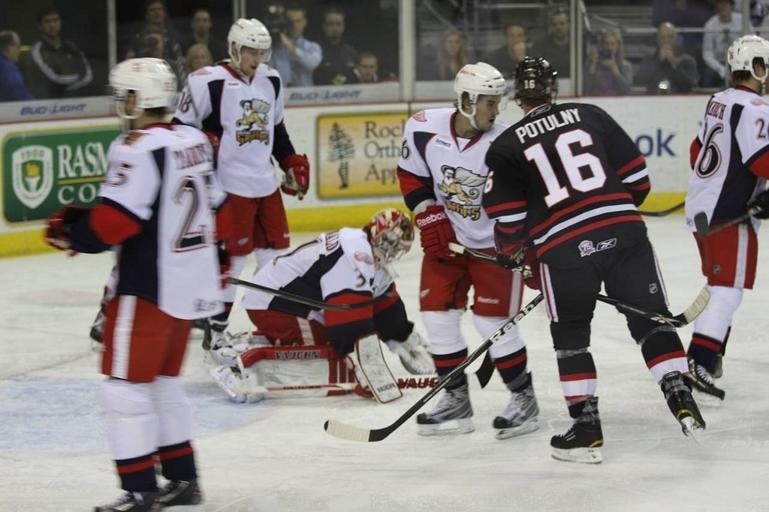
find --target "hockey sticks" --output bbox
[694,206,762,239]
[235,352,494,393]
[322,290,546,443]
[452,245,713,328]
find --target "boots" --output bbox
[491,371,542,441]
[208,328,242,363]
[657,370,711,449]
[416,373,478,437]
[208,355,273,405]
[547,395,605,464]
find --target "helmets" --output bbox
[107,56,180,110]
[726,33,769,82]
[226,17,273,68]
[364,207,415,265]
[513,55,559,100]
[453,61,506,119]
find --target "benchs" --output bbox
[417,0,659,87]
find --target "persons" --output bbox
[426,8,571,78]
[43,55,226,511]
[396,60,541,428]
[481,54,707,450]
[208,210,435,405]
[170,17,311,351]
[582,0,769,98]
[0,0,95,100]
[266,5,380,85]
[683,33,769,399]
[124,0,227,93]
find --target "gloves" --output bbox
[45,218,93,258]
[414,203,461,266]
[280,153,312,202]
[520,241,542,291]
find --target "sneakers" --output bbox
[682,356,727,407]
[153,477,203,511]
[93,487,163,511]
